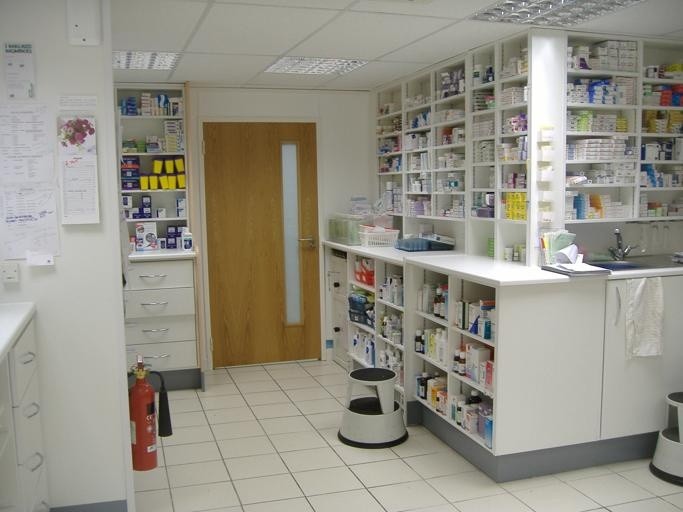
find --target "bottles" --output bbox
[351,328,404,396]
[477,309,491,340]
[648,201,668,217]
[431,288,448,318]
[413,330,466,375]
[415,371,482,433]
[382,314,402,345]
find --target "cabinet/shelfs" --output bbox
[604,266,683,469]
[0,302,48,512]
[114,82,206,392]
[372,30,530,265]
[564,26,682,256]
[324,234,601,485]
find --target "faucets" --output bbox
[607,227,632,260]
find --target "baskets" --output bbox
[360,229,400,248]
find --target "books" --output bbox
[557,262,605,271]
[540,263,611,277]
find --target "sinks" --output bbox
[584,260,643,272]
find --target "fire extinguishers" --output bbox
[127,355,172,471]
